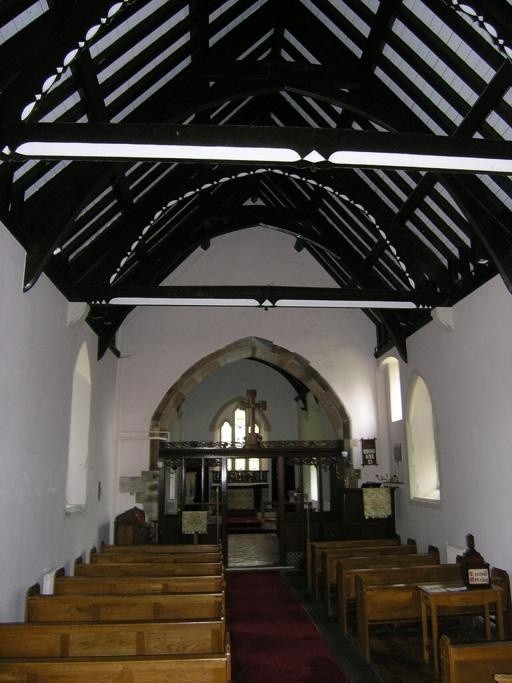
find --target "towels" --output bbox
[182,511,208,535]
[363,487,394,521]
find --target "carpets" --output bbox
[228,517,262,534]
[224,569,352,682]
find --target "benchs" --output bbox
[305,534,511,683]
[0,538,234,682]
